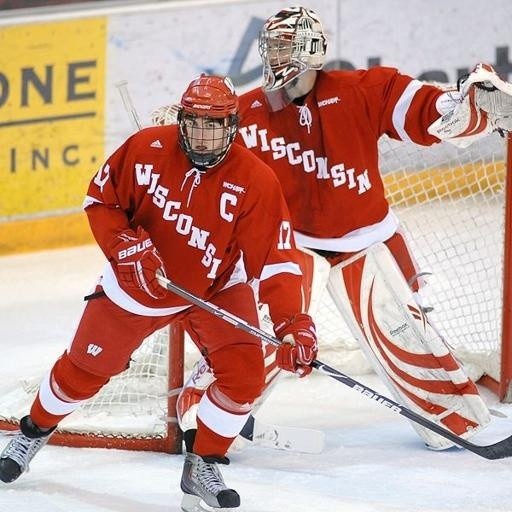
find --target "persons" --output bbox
[174,2,511,467]
[0,71,319,509]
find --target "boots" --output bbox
[0,414,59,483]
[179,428,241,509]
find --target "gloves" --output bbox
[271,312,320,380]
[105,225,170,302]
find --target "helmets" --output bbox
[256,6,329,94]
[176,71,241,170]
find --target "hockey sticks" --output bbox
[153,274,511,461]
